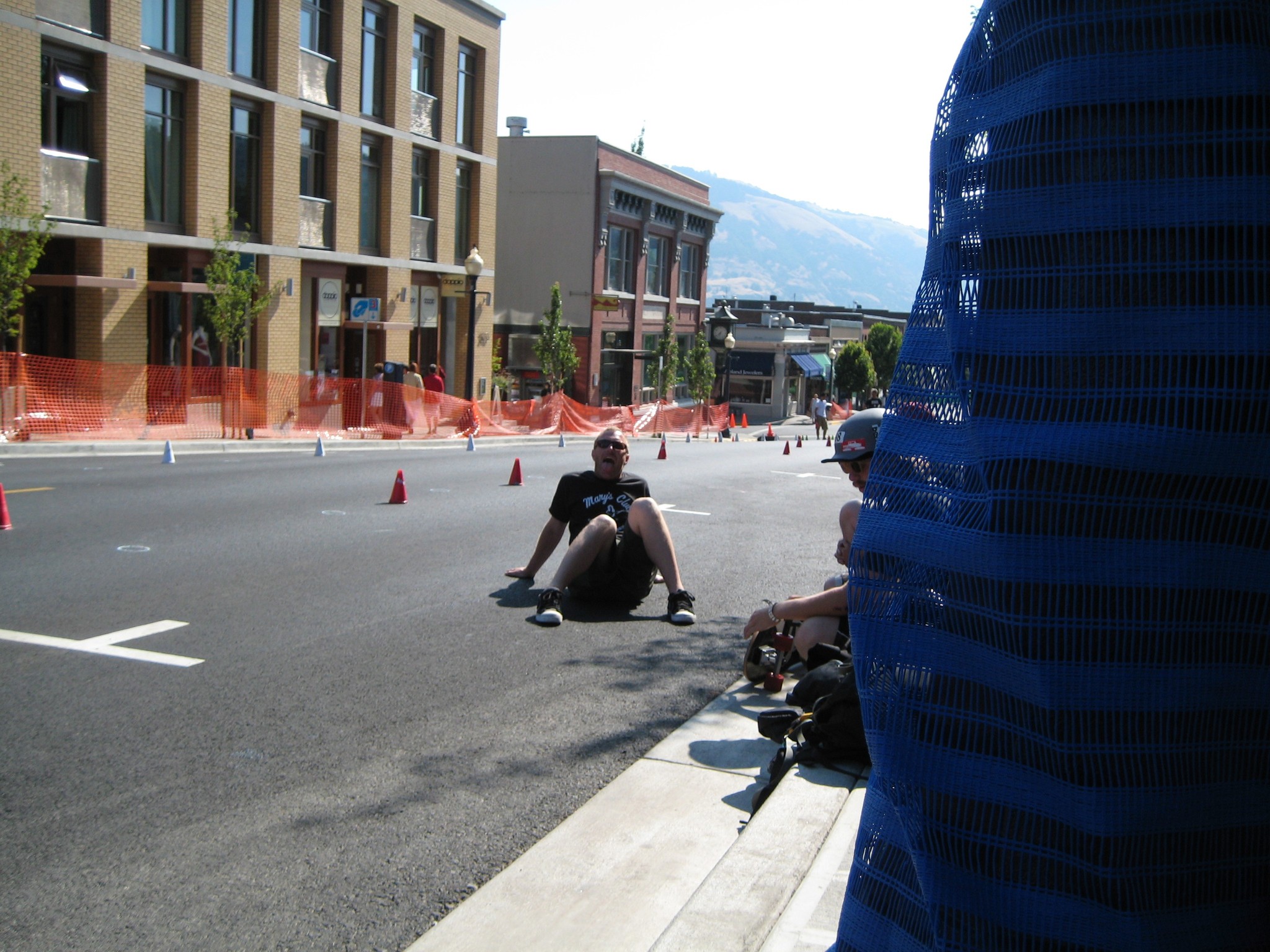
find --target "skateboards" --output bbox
[744,594,806,692]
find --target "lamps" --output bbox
[122,266,134,278]
[484,293,491,306]
[282,277,293,295]
[396,286,408,302]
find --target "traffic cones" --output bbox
[732,436,735,442]
[0,482,13,531]
[783,440,790,455]
[314,436,326,457]
[729,413,736,428]
[661,432,667,443]
[735,433,739,442]
[388,469,408,505]
[161,439,176,464]
[718,431,723,442]
[507,458,523,486]
[558,433,565,447]
[657,441,667,459]
[466,434,475,451]
[796,436,802,447]
[685,432,691,443]
[742,413,748,428]
[767,423,774,438]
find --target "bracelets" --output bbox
[768,602,785,623]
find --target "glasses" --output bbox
[837,458,874,474]
[594,439,629,454]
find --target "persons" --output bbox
[402,361,425,435]
[540,381,556,429]
[809,394,821,424]
[881,388,892,408]
[863,388,882,409]
[814,393,829,440]
[503,427,699,624]
[366,363,385,425]
[742,408,981,678]
[425,364,445,435]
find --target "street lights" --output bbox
[825,347,837,411]
[719,332,736,439]
[454,246,485,438]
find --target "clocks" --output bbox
[713,326,727,340]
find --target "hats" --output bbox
[819,407,890,463]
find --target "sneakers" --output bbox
[535,587,564,623]
[667,589,696,623]
